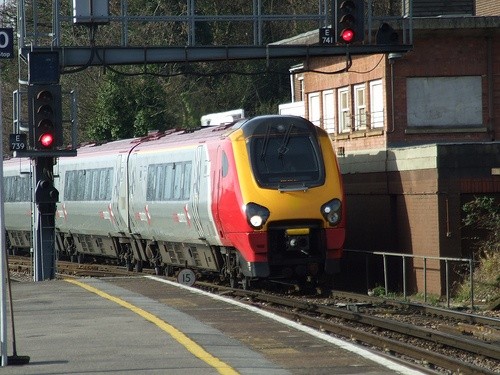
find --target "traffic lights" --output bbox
[335,0,364,43]
[28,83,63,148]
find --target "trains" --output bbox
[3,113,348,296]
